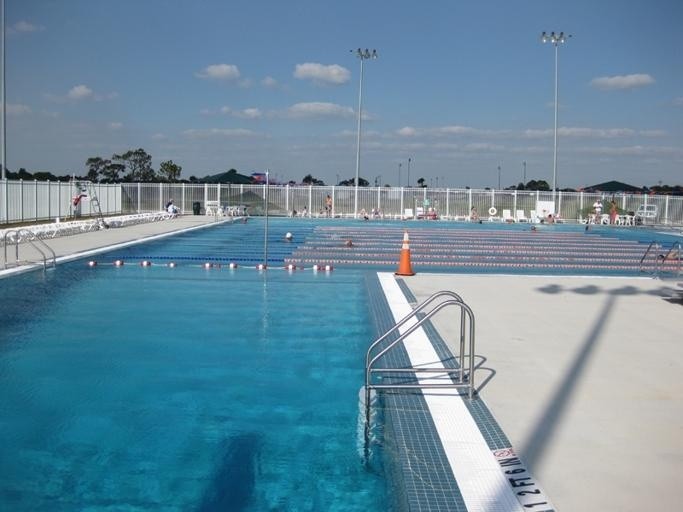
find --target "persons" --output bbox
[165,199,176,212]
[593,199,603,224]
[609,200,616,224]
[284,232,295,242]
[469,206,481,221]
[324,195,332,217]
[546,214,555,224]
[168,204,178,213]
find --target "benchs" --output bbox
[0,211,182,246]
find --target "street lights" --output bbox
[348,47,378,216]
[537,29,572,215]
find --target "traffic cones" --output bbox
[393,229,416,276]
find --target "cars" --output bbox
[634,205,660,223]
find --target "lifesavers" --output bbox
[488,207,497,215]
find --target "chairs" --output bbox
[204,205,251,217]
[502,209,540,223]
[588,213,635,226]
[404,207,436,220]
